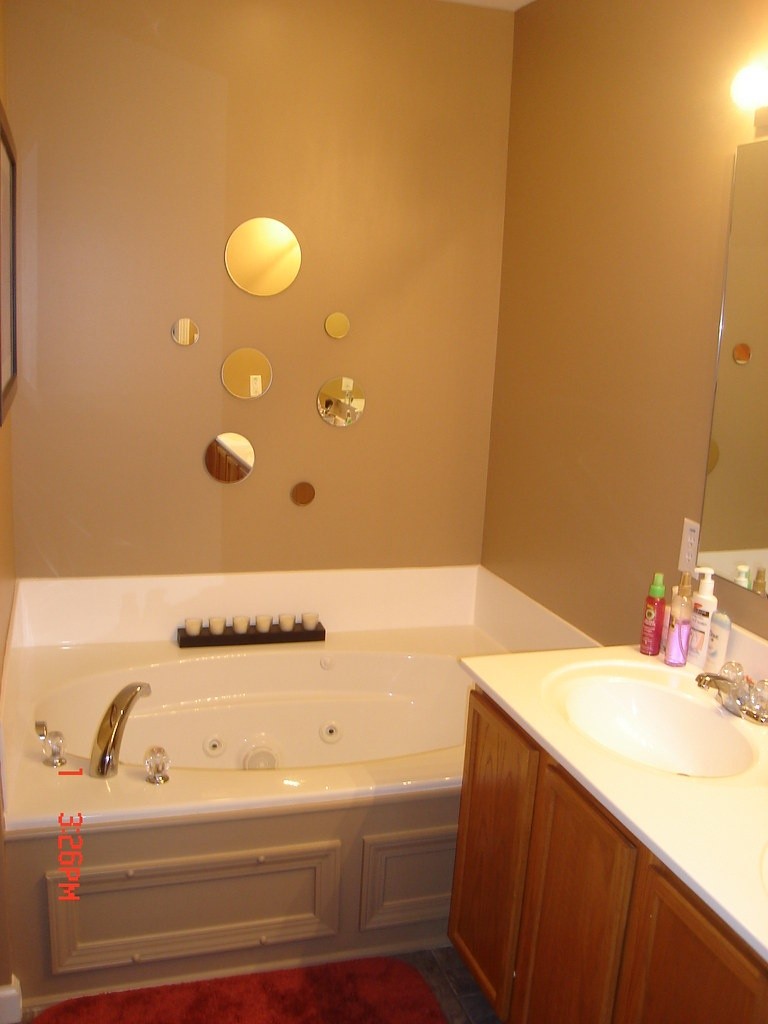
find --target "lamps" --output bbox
[730,62,768,128]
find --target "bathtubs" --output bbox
[0,624,604,1009]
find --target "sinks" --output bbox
[547,660,760,779]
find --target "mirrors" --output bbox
[695,138,768,597]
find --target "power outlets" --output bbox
[678,518,701,580]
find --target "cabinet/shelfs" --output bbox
[443,682,768,1024]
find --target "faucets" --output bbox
[696,673,741,718]
[88,682,151,779]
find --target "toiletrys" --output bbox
[734,564,765,596]
[640,567,731,673]
